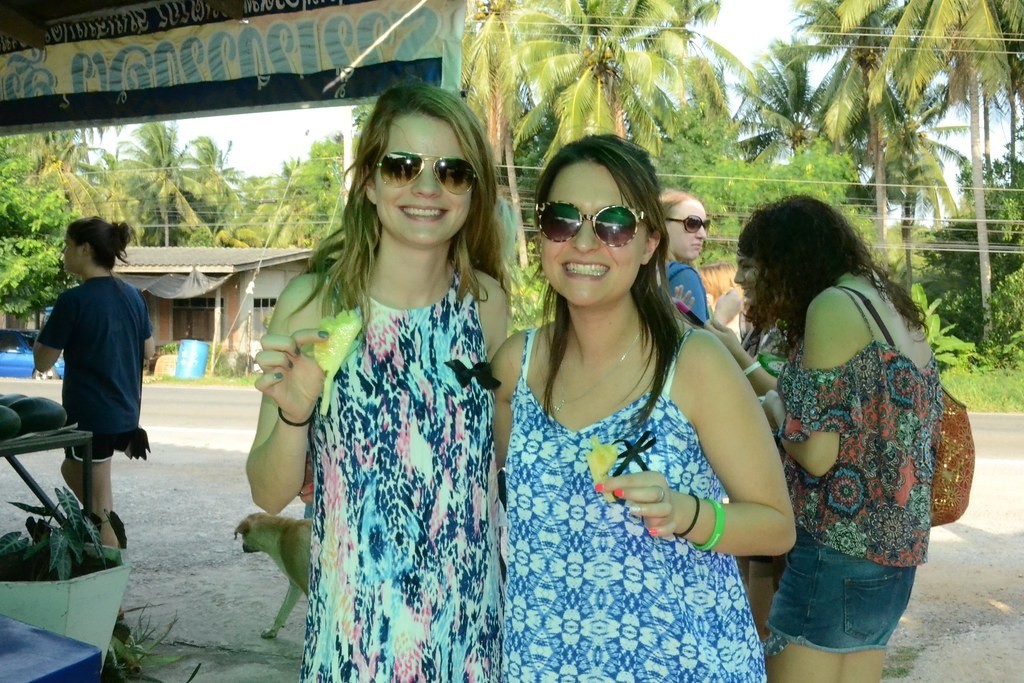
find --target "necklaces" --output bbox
[552,330,640,415]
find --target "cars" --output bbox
[0,329,64,380]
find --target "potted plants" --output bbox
[0,486,133,675]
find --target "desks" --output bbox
[0,430,94,541]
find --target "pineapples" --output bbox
[586,434,618,503]
[313,309,362,415]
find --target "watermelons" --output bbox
[0,394,67,442]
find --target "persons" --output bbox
[490,133,796,683]
[704,251,803,643]
[737,194,943,683]
[438,162,465,187]
[246,80,507,683]
[383,156,412,182]
[32,216,155,645]
[656,190,744,329]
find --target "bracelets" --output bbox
[277,406,315,427]
[672,493,700,537]
[692,499,725,551]
[743,361,761,376]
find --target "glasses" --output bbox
[376,151,478,195]
[534,201,645,248]
[666,214,711,233]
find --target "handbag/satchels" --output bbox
[930,382,975,527]
[125,428,151,461]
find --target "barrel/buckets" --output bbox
[174,338,210,381]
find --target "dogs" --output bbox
[233,512,311,639]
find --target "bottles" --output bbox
[756,319,790,378]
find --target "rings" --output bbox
[654,485,665,503]
